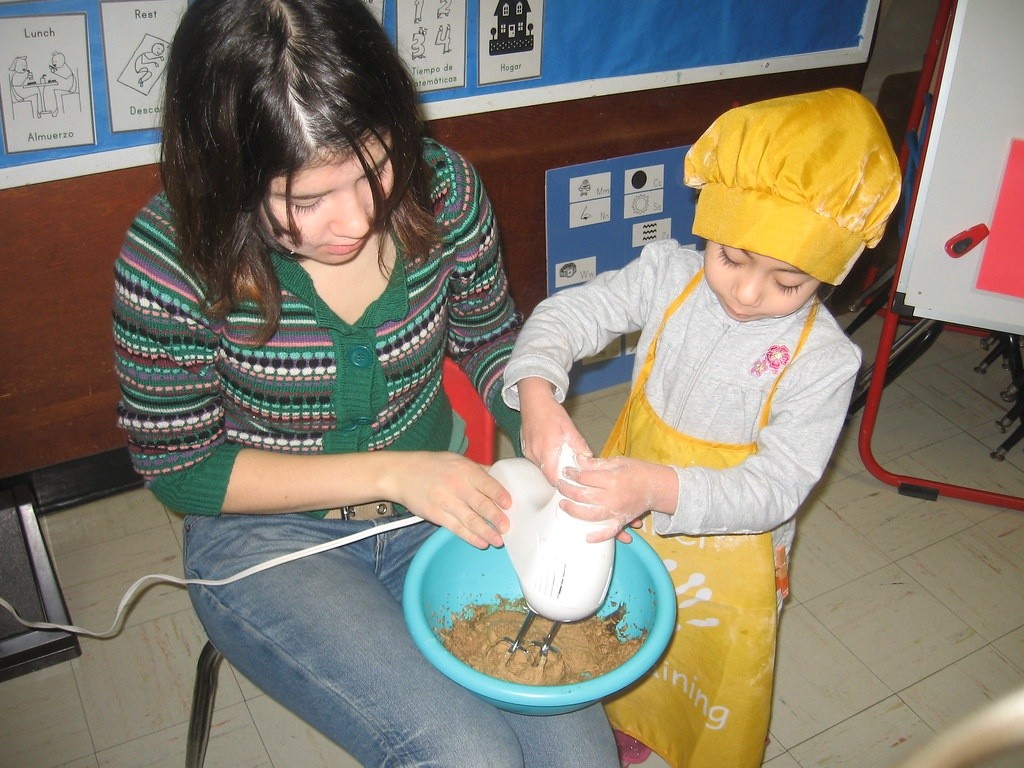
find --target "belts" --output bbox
[323,502,408,521]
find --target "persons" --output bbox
[112,0,640,768]
[500,86,903,768]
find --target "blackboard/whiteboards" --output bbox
[890,1,1024,343]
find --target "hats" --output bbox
[683,89,901,286]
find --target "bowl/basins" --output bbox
[402,525,677,717]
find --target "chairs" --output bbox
[846,0,1023,461]
[184,354,495,768]
